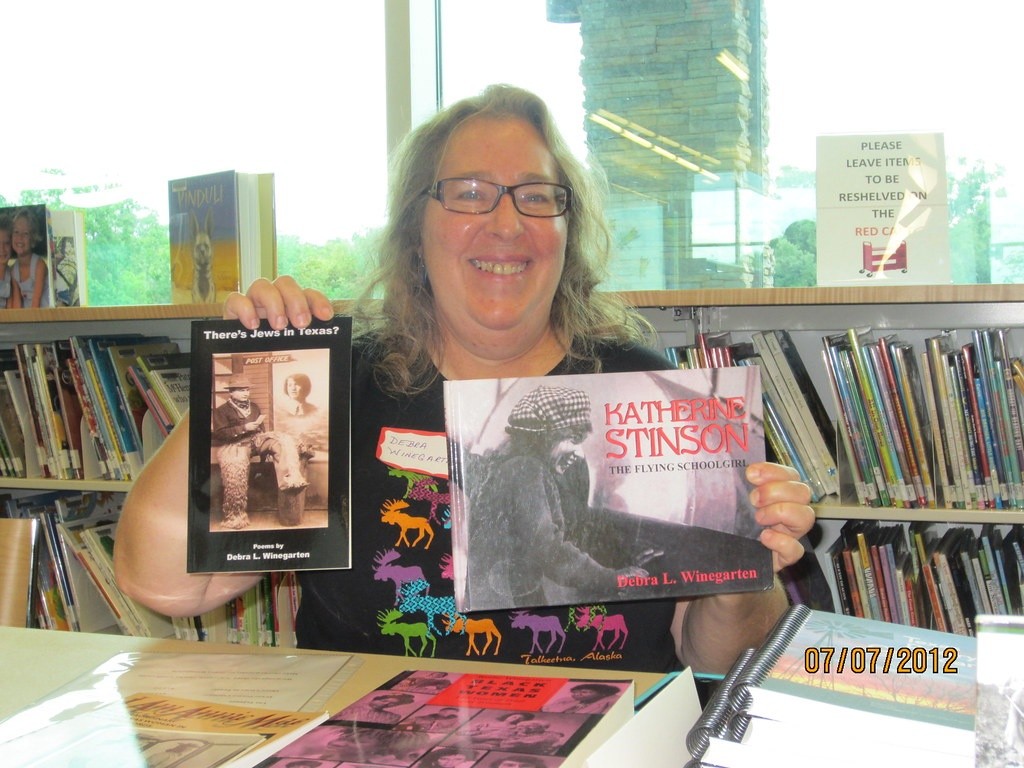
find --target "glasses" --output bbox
[423,177,573,218]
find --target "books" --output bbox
[817,133,949,286]
[186,317,353,572]
[0,333,301,646]
[0,203,54,308]
[51,210,87,307]
[442,365,776,614]
[168,170,277,304]
[0,693,330,768]
[252,669,634,768]
[663,328,1024,634]
[679,606,979,768]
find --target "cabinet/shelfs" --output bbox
[0,284,1024,644]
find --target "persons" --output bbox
[215,373,317,526]
[114,85,815,676]
[282,670,620,768]
[467,385,649,609]
[0,209,49,308]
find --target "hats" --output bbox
[223,376,254,388]
[504,386,592,437]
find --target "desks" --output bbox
[0,624,672,768]
